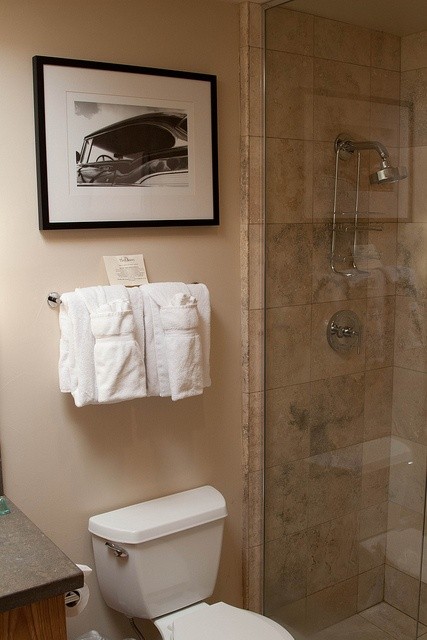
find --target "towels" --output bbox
[142,281,212,401]
[58,285,148,407]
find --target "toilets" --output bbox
[305,435,427,584]
[89,486,294,639]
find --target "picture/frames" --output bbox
[30,54,220,230]
